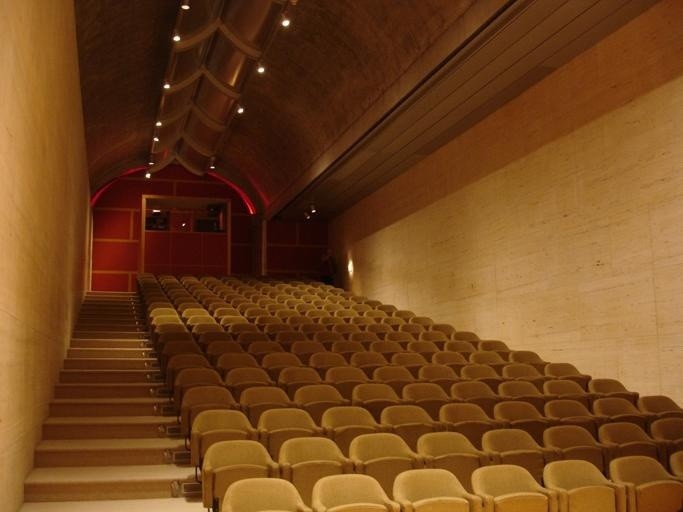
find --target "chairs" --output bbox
[129,271,682,512]
